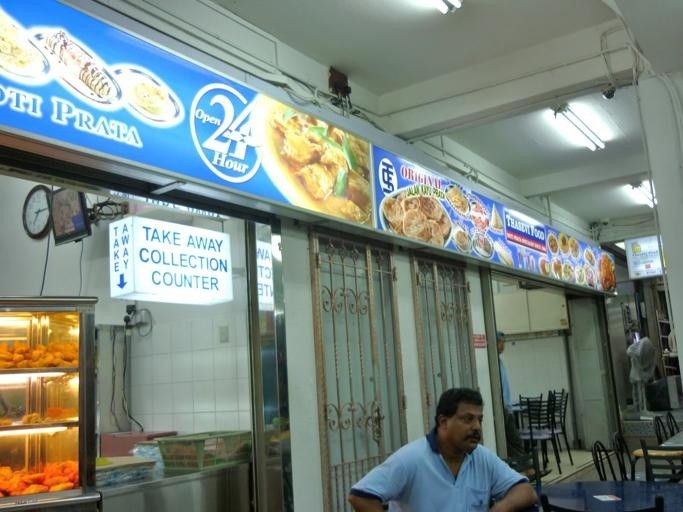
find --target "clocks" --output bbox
[22,185,54,239]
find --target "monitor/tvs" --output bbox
[46,188,91,246]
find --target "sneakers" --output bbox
[527,469,552,483]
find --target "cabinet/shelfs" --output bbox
[0,293,104,505]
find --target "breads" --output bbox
[0,342,79,497]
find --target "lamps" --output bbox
[557,103,658,212]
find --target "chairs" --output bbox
[512,388,683,512]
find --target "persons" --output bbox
[347,388,538,512]
[495,330,553,481]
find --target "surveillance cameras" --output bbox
[601,87,616,99]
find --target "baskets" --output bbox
[153,431,252,471]
